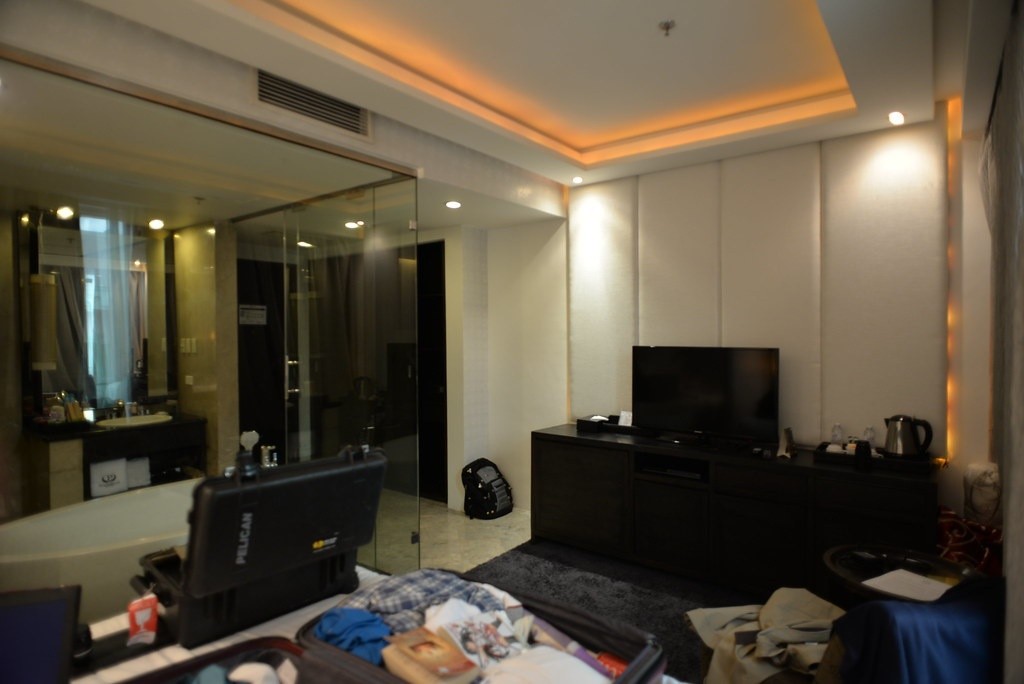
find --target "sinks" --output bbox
[96,415,173,428]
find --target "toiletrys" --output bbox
[137,400,144,417]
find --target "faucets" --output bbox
[261,446,276,468]
[111,400,125,417]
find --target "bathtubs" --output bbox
[1,476,303,627]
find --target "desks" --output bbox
[21,414,208,520]
[531,423,948,600]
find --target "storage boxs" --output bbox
[128,443,387,651]
[112,567,667,684]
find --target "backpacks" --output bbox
[460,457,514,520]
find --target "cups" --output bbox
[41,578,94,658]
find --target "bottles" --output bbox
[832,423,842,442]
[865,425,875,448]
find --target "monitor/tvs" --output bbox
[632,347,780,459]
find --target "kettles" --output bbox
[882,414,933,463]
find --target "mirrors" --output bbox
[28,210,176,419]
